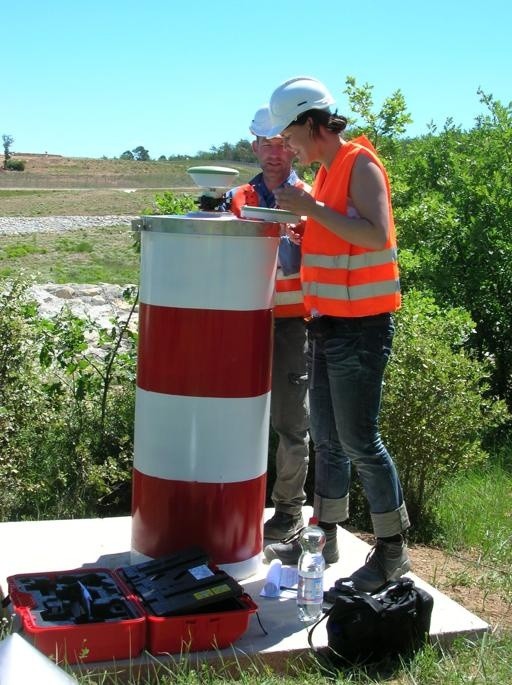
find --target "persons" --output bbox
[267,74,417,594]
[216,105,314,543]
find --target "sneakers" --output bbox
[264,511,304,540]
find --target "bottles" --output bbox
[295,516,327,624]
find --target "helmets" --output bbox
[249,106,285,139]
[266,78,335,140]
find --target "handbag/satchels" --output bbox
[327,577,434,676]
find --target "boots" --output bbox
[263,521,338,565]
[349,532,412,591]
